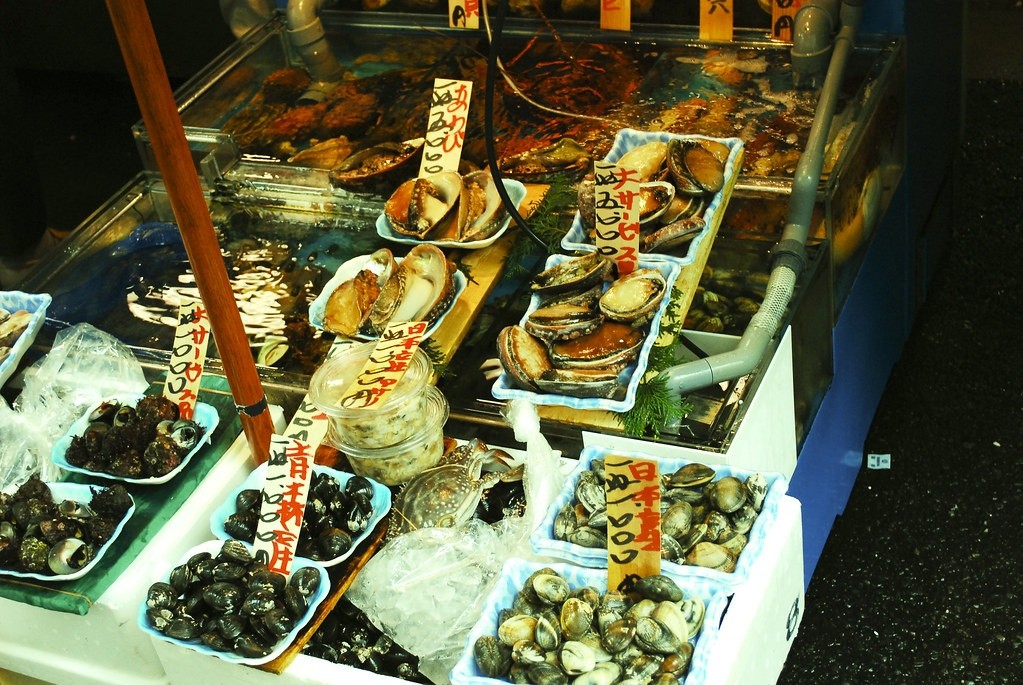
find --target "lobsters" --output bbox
[450,0,639,154]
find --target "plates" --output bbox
[0,482,135,580]
[447,442,790,685]
[209,461,391,567]
[330,137,425,182]
[560,127,744,266]
[309,255,467,344]
[49,393,220,484]
[375,178,526,249]
[137,540,330,666]
[492,254,681,415]
[0,290,52,388]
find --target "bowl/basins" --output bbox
[309,344,448,487]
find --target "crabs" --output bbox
[385,437,524,543]
